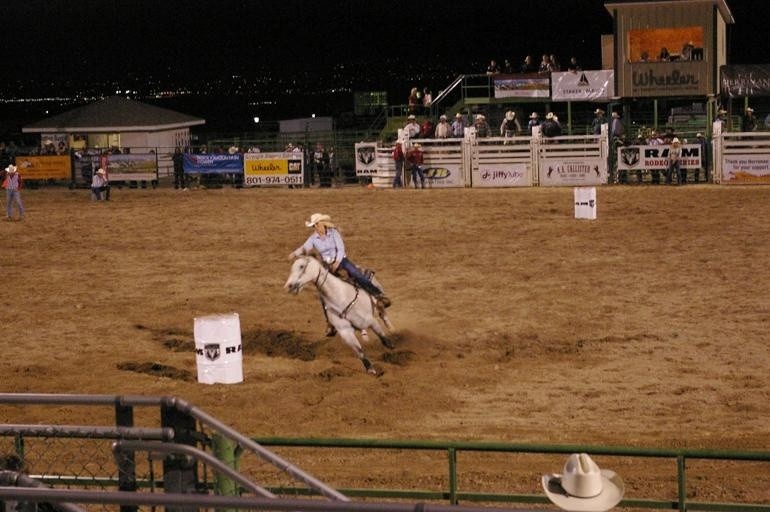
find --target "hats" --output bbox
[610,111,621,119]
[5,165,17,173]
[228,146,239,155]
[670,137,681,143]
[505,111,515,120]
[529,112,554,119]
[305,213,331,227]
[394,114,422,148]
[594,108,604,114]
[439,112,485,119]
[96,168,105,175]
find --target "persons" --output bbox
[500,111,522,145]
[0,164,24,222]
[743,108,757,141]
[90,168,114,203]
[423,87,432,115]
[528,112,559,137]
[287,213,391,337]
[392,140,404,188]
[171,141,334,191]
[615,130,707,186]
[406,115,491,146]
[591,109,608,142]
[716,109,727,132]
[487,52,581,75]
[612,111,622,137]
[638,38,697,63]
[409,88,418,116]
[410,143,425,189]
[0,139,158,189]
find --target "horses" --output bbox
[283,243,401,374]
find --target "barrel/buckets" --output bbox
[194,312,243,384]
[574,186,597,220]
